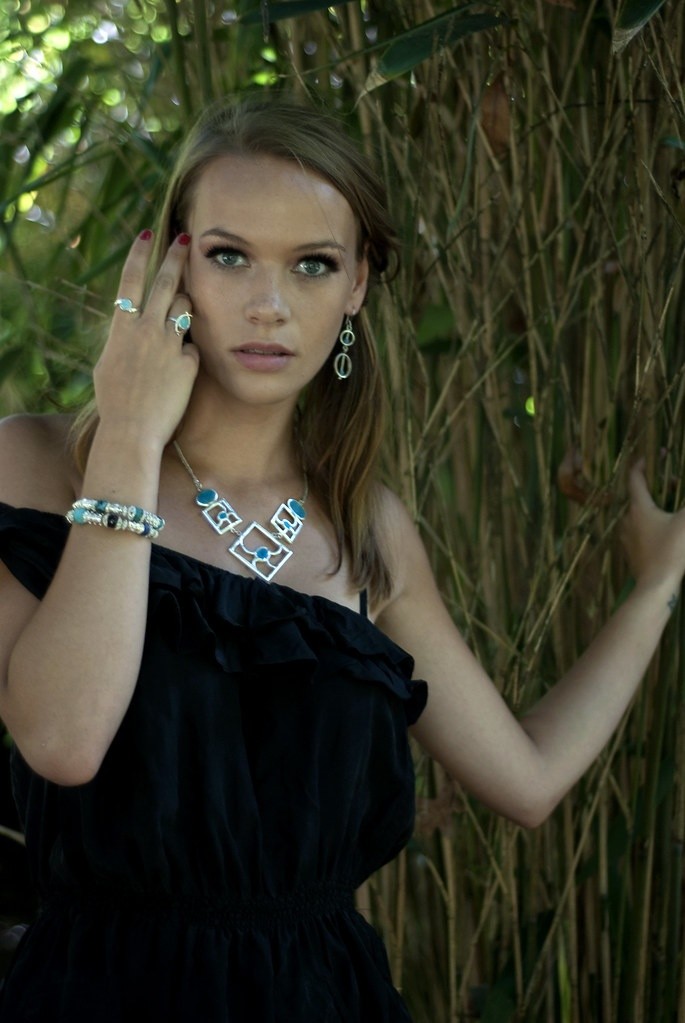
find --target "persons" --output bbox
[0,93,685,1023]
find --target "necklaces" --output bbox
[171,437,308,583]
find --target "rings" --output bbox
[166,311,194,338]
[114,297,141,313]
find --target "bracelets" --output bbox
[66,498,165,539]
